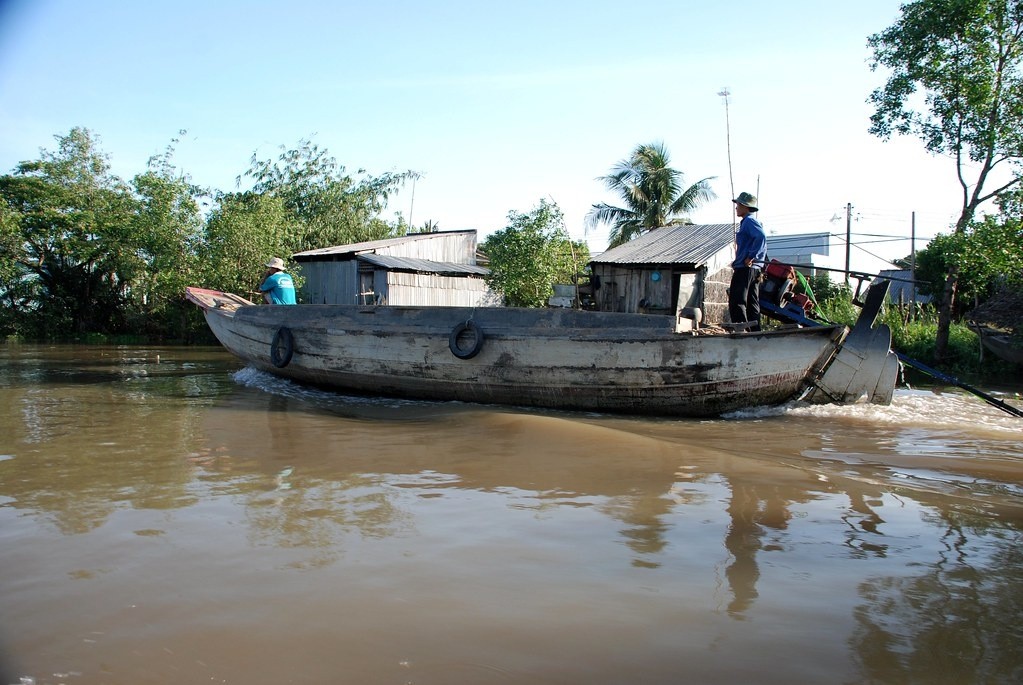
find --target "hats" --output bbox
[732,192,759,212]
[264,257,287,270]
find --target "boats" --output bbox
[187,226,901,409]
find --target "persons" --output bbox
[259,256,297,305]
[728,192,767,333]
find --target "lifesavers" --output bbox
[448,319,485,359]
[271,326,294,369]
